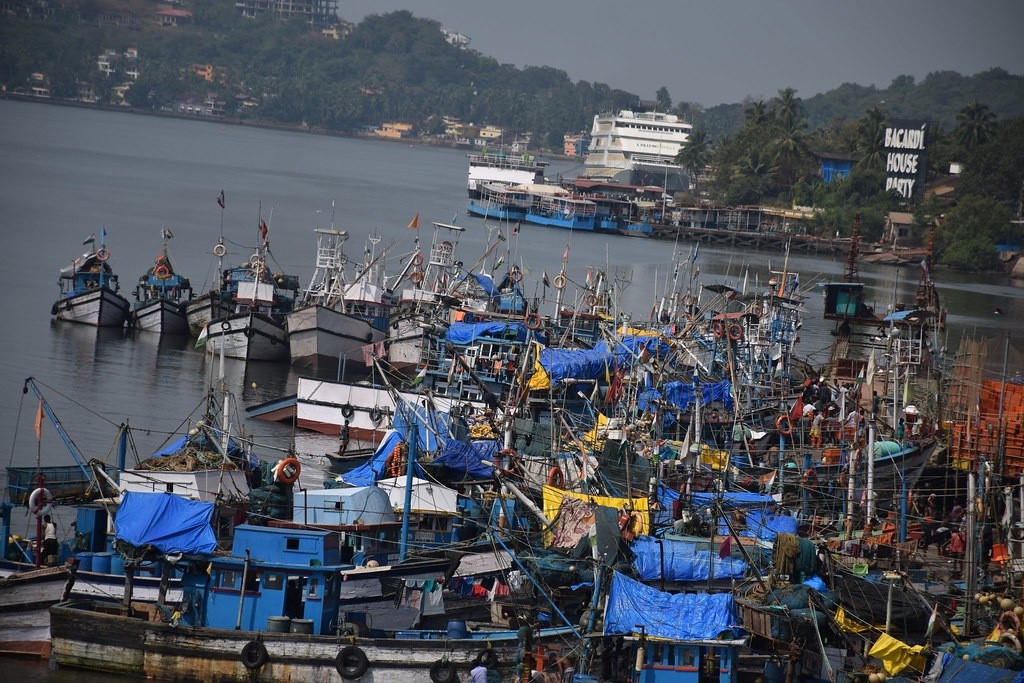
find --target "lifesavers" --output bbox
[728,323,741,340]
[367,332,373,341]
[681,293,695,307]
[51,301,59,315]
[270,335,278,345]
[494,448,520,475]
[341,403,475,421]
[750,305,763,319]
[156,266,169,278]
[241,641,498,683]
[28,487,53,517]
[393,321,398,329]
[213,244,227,257]
[632,513,643,542]
[777,414,912,509]
[510,269,601,330]
[998,611,1022,655]
[277,456,303,485]
[548,466,564,490]
[249,253,264,279]
[221,320,231,330]
[243,326,255,338]
[713,320,723,340]
[410,240,452,283]
[65,299,72,311]
[97,248,111,261]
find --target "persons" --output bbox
[40,514,59,562]
[471,659,487,683]
[925,493,966,572]
[802,376,879,448]
[514,621,532,683]
[749,439,756,463]
[769,445,779,467]
[549,653,574,683]
[898,418,905,441]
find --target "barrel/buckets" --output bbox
[289,618,314,634]
[78,551,190,579]
[268,616,291,633]
[447,618,466,639]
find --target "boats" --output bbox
[466,127,655,240]
[1,190,1023,682]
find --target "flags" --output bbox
[720,538,730,560]
